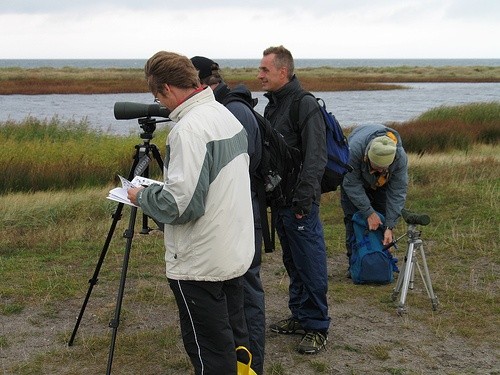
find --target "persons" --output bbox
[127,52,266,375]
[340,122,408,280]
[258,47,332,355]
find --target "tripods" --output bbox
[67,117,165,375]
[382,224,439,311]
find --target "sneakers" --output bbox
[268,317,305,335]
[296,329,329,355]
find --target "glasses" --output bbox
[154,90,161,104]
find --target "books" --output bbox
[106,174,164,207]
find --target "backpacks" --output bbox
[221,95,302,210]
[290,90,353,194]
[346,209,399,285]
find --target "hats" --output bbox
[191,56,220,79]
[368,135,397,168]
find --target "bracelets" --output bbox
[385,226,393,230]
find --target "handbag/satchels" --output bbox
[236,345,258,375]
